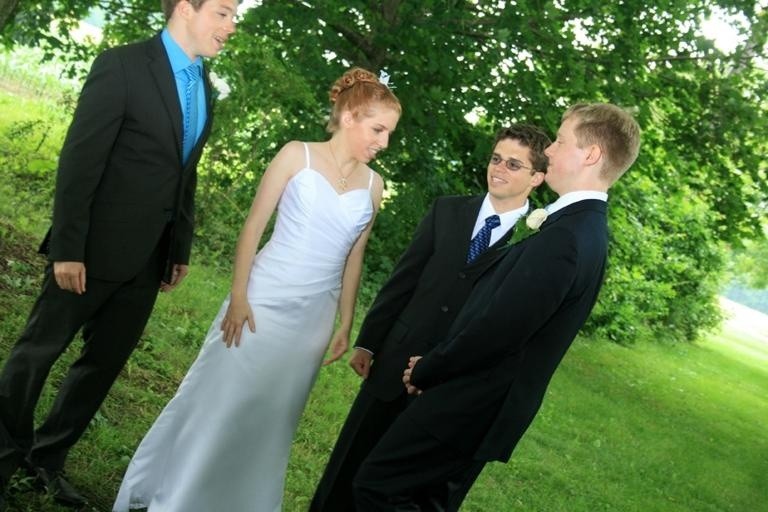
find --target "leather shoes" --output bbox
[23,462,86,507]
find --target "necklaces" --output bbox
[329,148,360,193]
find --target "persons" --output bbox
[0,1,244,506]
[305,123,554,511]
[107,69,402,512]
[353,100,634,512]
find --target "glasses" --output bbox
[485,153,536,172]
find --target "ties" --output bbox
[182,63,200,162]
[467,215,500,263]
